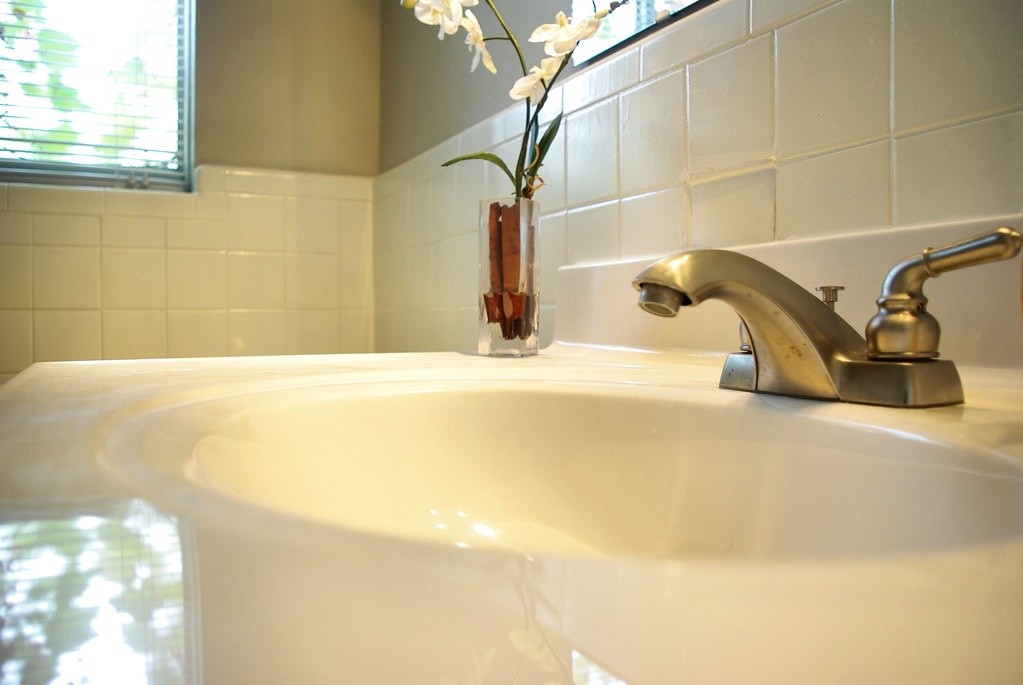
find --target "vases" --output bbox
[480,198,540,357]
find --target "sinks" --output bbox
[1,340,1023,683]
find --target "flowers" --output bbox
[399,0,629,202]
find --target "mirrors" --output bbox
[570,0,704,66]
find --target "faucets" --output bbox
[629,227,1017,409]
